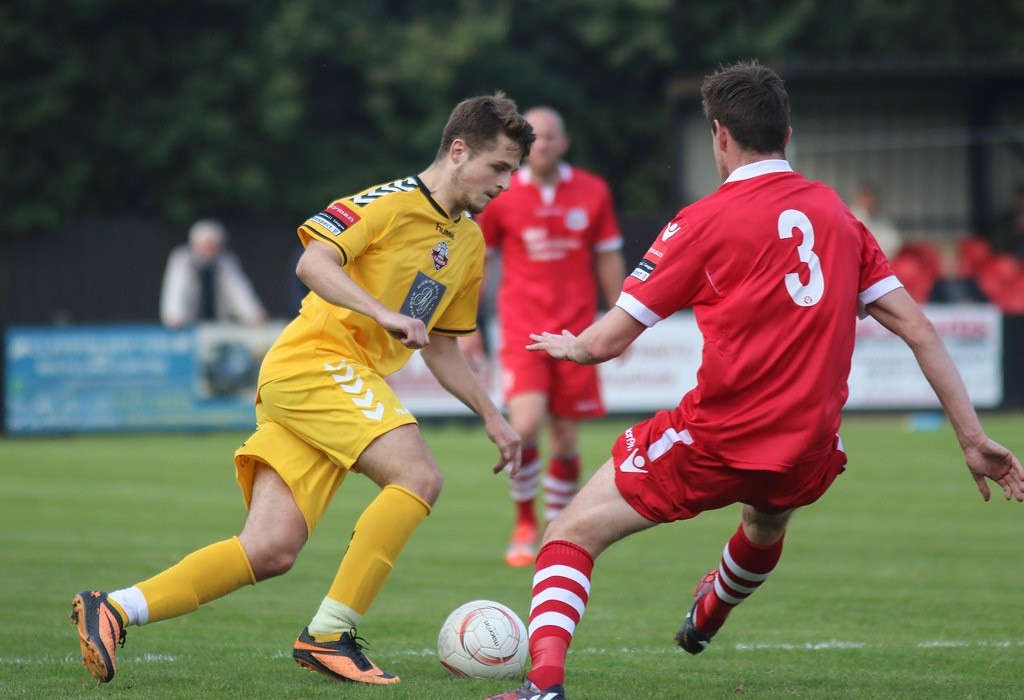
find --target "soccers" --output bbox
[434,599,531,680]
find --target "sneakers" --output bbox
[506,517,536,565]
[483,670,566,700]
[71,591,123,687]
[293,626,400,686]
[675,569,719,656]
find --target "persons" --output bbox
[489,60,1024,700]
[956,183,1023,408]
[160,220,265,324]
[477,107,633,569]
[70,95,522,686]
[850,181,949,303]
